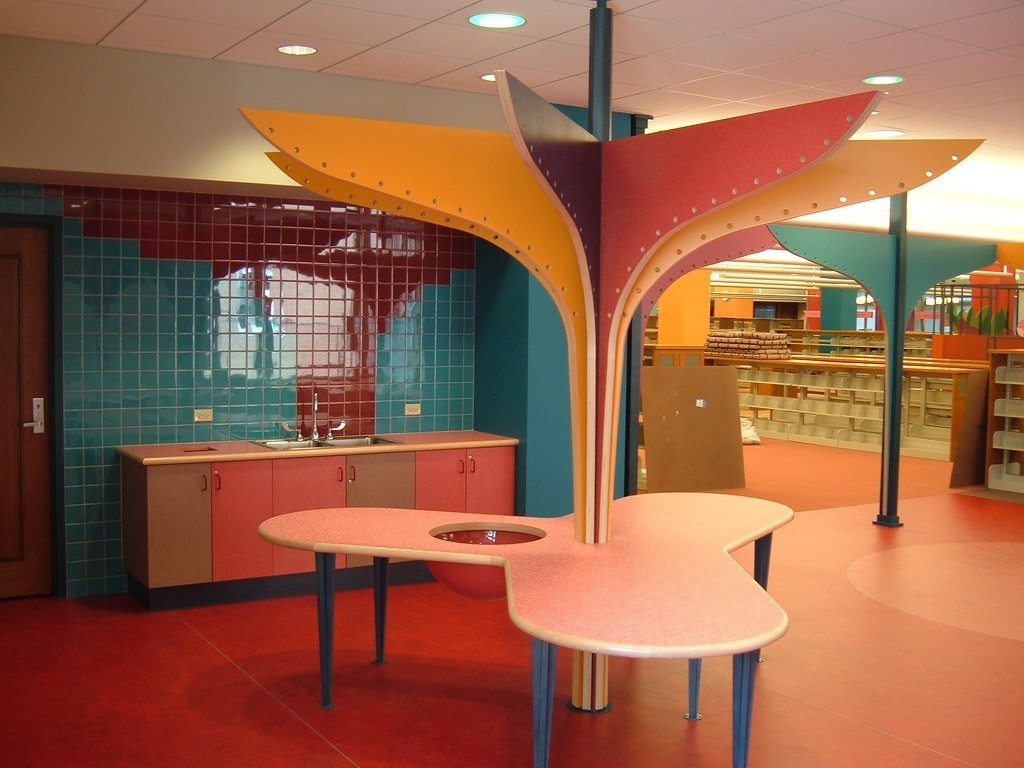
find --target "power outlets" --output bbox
[404,403,421,415]
[194,409,213,422]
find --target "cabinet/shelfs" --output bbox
[115,431,519,602]
[636,316,1024,494]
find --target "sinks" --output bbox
[320,435,405,449]
[247,439,328,450]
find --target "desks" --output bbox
[256,493,795,768]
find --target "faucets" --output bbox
[312,393,319,439]
